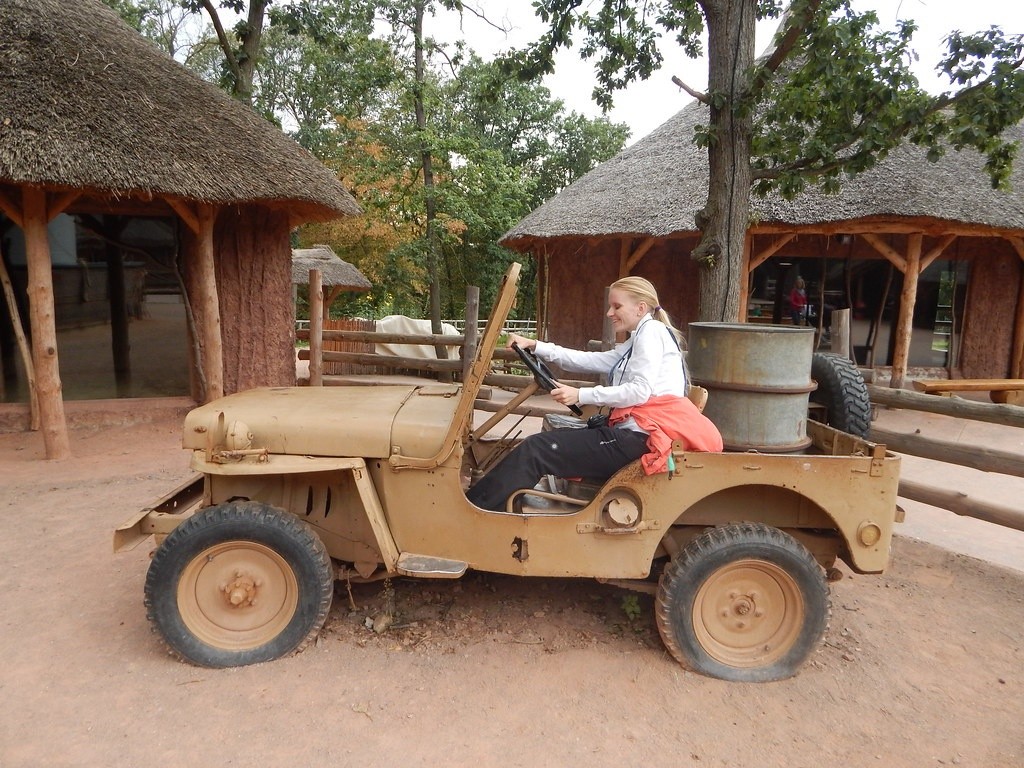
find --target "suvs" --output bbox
[109,261,907,686]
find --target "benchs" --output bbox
[542,383,708,502]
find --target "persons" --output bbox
[789,274,809,324]
[466,277,692,511]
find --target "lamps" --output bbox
[838,234,850,243]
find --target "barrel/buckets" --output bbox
[689,321,818,453]
